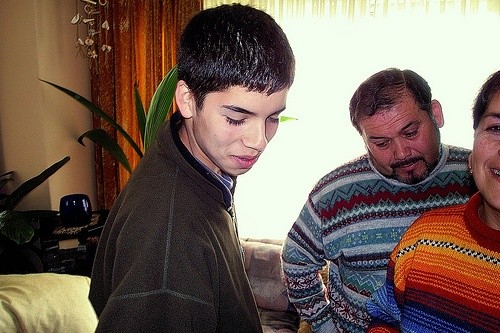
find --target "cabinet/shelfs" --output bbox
[37,209,111,276]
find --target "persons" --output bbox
[88,3,295,333]
[281,68,474,333]
[364,70,500,333]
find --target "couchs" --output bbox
[242,238,328,333]
